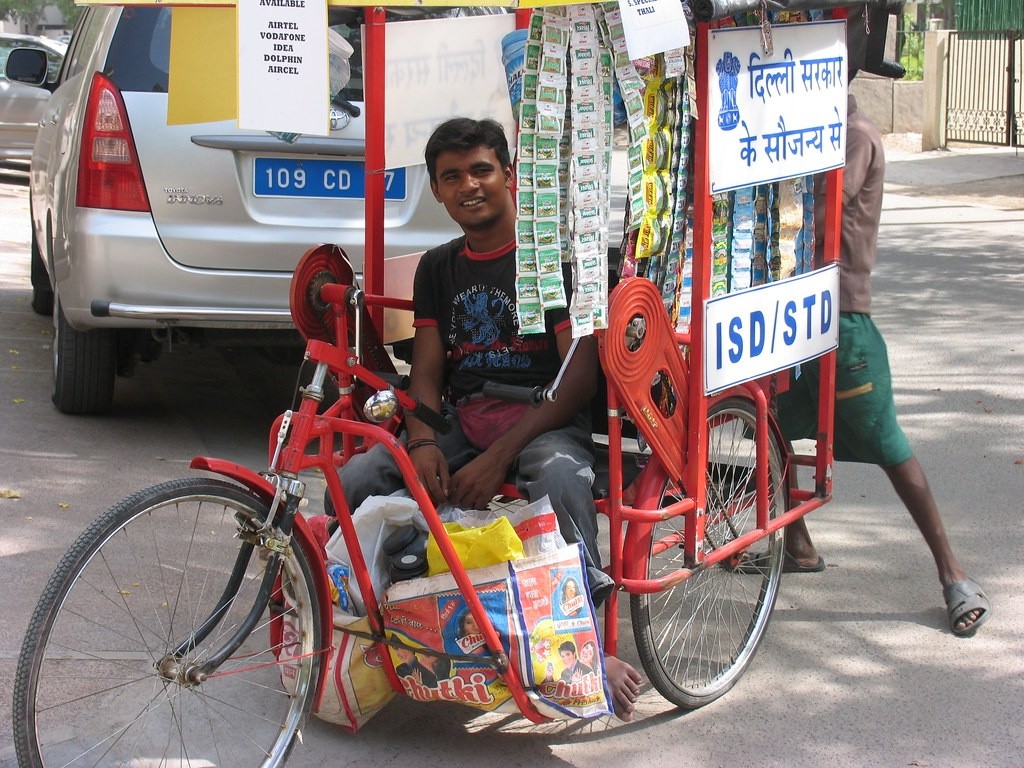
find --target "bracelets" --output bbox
[405,438,439,453]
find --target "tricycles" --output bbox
[12,7,849,767]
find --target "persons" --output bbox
[717,61,991,636]
[324,117,642,722]
[562,577,582,616]
[389,634,438,688]
[542,641,598,684]
[417,648,451,680]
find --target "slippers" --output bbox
[746,549,826,573]
[942,576,993,636]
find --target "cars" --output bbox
[0,32,70,162]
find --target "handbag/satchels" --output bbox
[456,391,527,451]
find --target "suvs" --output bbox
[8,3,533,419]
